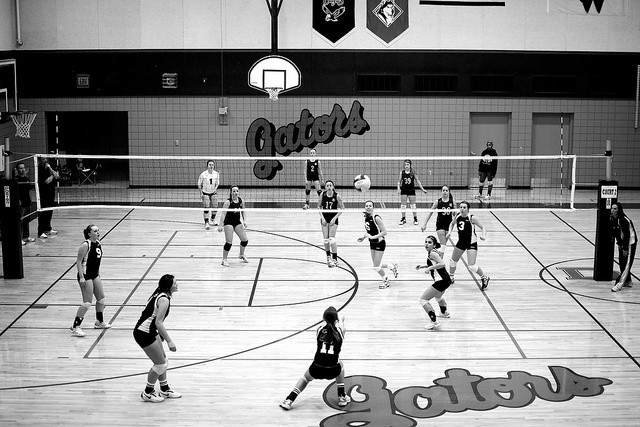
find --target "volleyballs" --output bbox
[353,173,371,192]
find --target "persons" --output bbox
[420,184,458,259]
[68,224,112,337]
[278,307,352,410]
[415,236,453,330]
[36,155,59,238]
[397,160,428,225]
[132,274,183,403]
[196,159,220,229]
[302,148,325,209]
[610,202,638,292]
[445,201,491,290]
[76,153,86,170]
[357,200,399,289]
[475,140,498,200]
[221,185,249,266]
[317,180,346,266]
[12,162,36,245]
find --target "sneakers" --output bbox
[338,394,352,407]
[485,193,492,200]
[398,218,406,225]
[302,204,309,210]
[436,313,451,318]
[392,263,398,278]
[220,259,230,266]
[238,255,249,263]
[473,194,481,199]
[47,230,57,235]
[333,258,338,267]
[610,284,623,292]
[205,223,210,230]
[279,399,293,410]
[423,321,441,330]
[378,283,390,289]
[21,240,27,245]
[24,237,36,242]
[480,276,491,292]
[69,326,87,337]
[159,389,183,398]
[625,280,633,287]
[413,220,419,225]
[94,320,112,329]
[450,276,454,284]
[211,219,218,226]
[39,232,48,238]
[327,260,333,268]
[140,390,165,403]
